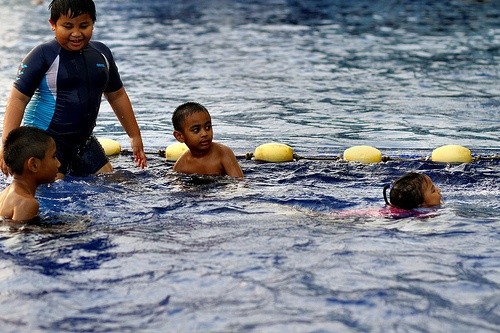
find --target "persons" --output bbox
[382,173,442,207]
[0,126,62,220]
[0,0,147,179]
[172,102,243,176]
